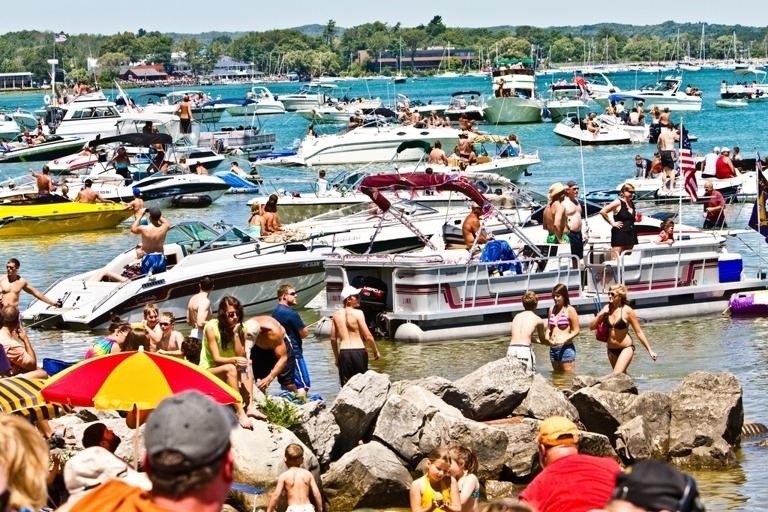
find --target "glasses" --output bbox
[573,187,579,190]
[627,190,635,194]
[7,265,14,269]
[286,292,295,296]
[608,292,614,296]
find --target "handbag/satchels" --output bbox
[596,322,609,342]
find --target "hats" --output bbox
[713,147,731,152]
[549,180,579,196]
[144,389,238,473]
[184,95,191,101]
[538,415,581,447]
[340,285,363,300]
[85,179,94,184]
[457,132,468,136]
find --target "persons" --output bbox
[22,80,744,274]
[1,258,707,511]
